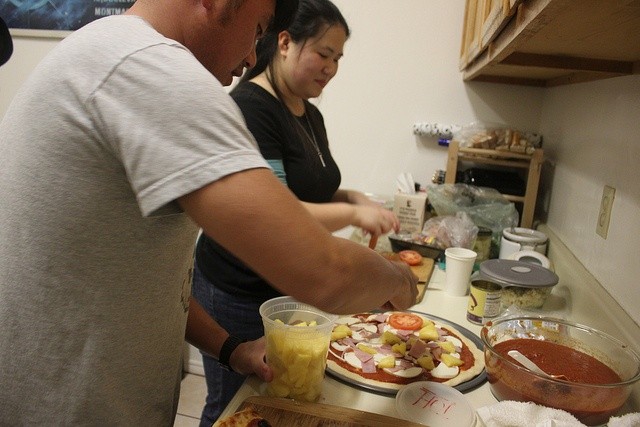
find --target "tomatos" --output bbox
[389,313,424,330]
[399,250,422,265]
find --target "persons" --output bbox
[0,0,428,426]
[189,0,403,427]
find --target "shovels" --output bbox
[507,349,572,392]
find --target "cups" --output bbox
[259,294,332,404]
[444,247,477,297]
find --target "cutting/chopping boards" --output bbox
[235,396,430,427]
[410,257,434,305]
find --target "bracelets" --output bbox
[218,328,243,372]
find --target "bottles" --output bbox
[431,168,526,196]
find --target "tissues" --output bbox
[392,172,427,234]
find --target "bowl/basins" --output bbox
[481,315,640,427]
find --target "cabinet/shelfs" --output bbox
[445,140,546,231]
[458,0,640,88]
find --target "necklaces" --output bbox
[281,96,328,167]
